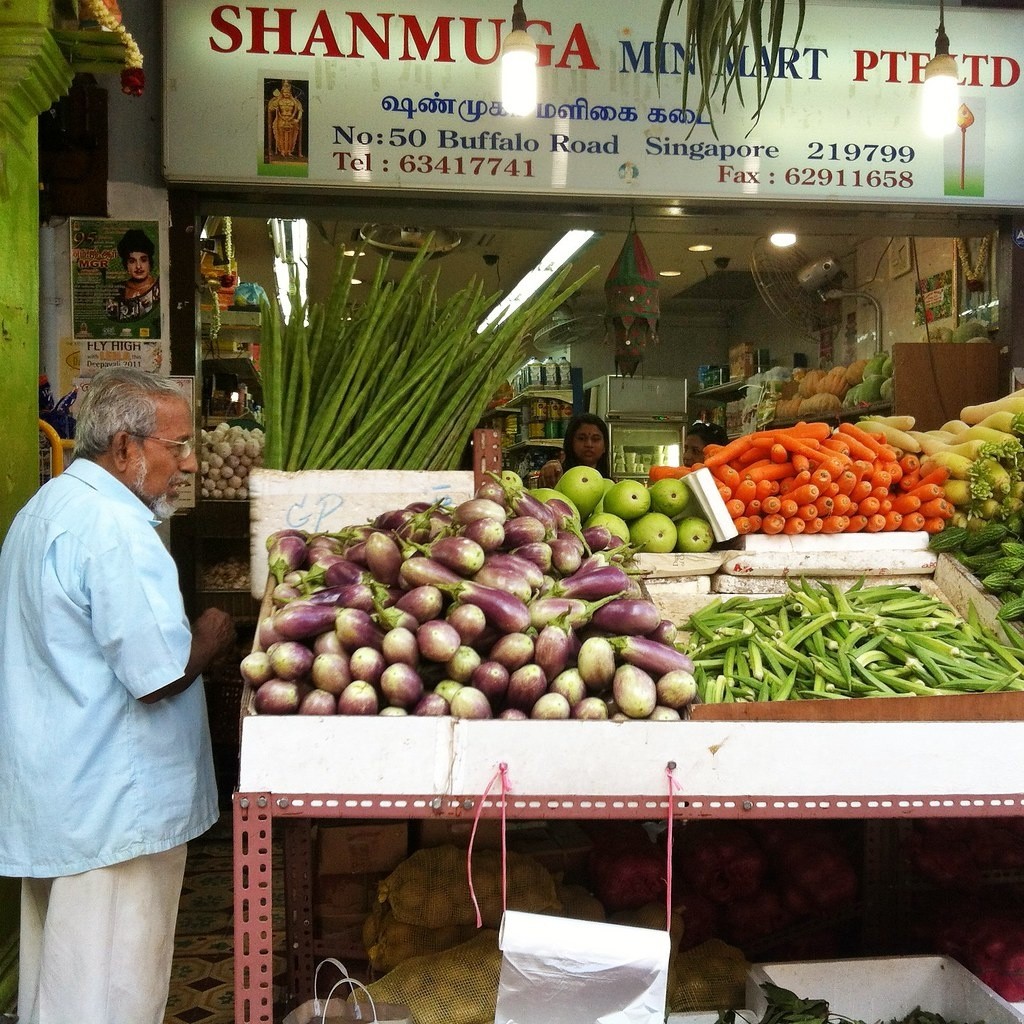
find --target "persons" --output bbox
[680,421,729,468]
[1,364,238,1024]
[115,228,154,297]
[535,413,610,490]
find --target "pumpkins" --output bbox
[772,322,991,420]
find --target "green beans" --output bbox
[256,223,602,472]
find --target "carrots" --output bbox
[646,421,956,535]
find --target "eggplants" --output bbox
[239,471,698,722]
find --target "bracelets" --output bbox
[546,459,560,464]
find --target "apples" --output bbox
[577,813,1024,1003]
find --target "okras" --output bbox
[675,572,1024,704]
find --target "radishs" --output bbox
[855,386,1024,530]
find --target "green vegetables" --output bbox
[714,983,989,1024]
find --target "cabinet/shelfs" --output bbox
[192,307,266,746]
[760,343,1011,432]
[687,377,748,444]
[460,367,583,490]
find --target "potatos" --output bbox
[350,846,751,1024]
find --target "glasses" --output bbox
[125,429,193,459]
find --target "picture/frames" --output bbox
[915,270,953,325]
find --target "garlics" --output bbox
[195,424,265,500]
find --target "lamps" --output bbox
[921,0,959,139]
[269,218,309,328]
[476,229,594,334]
[501,0,538,116]
